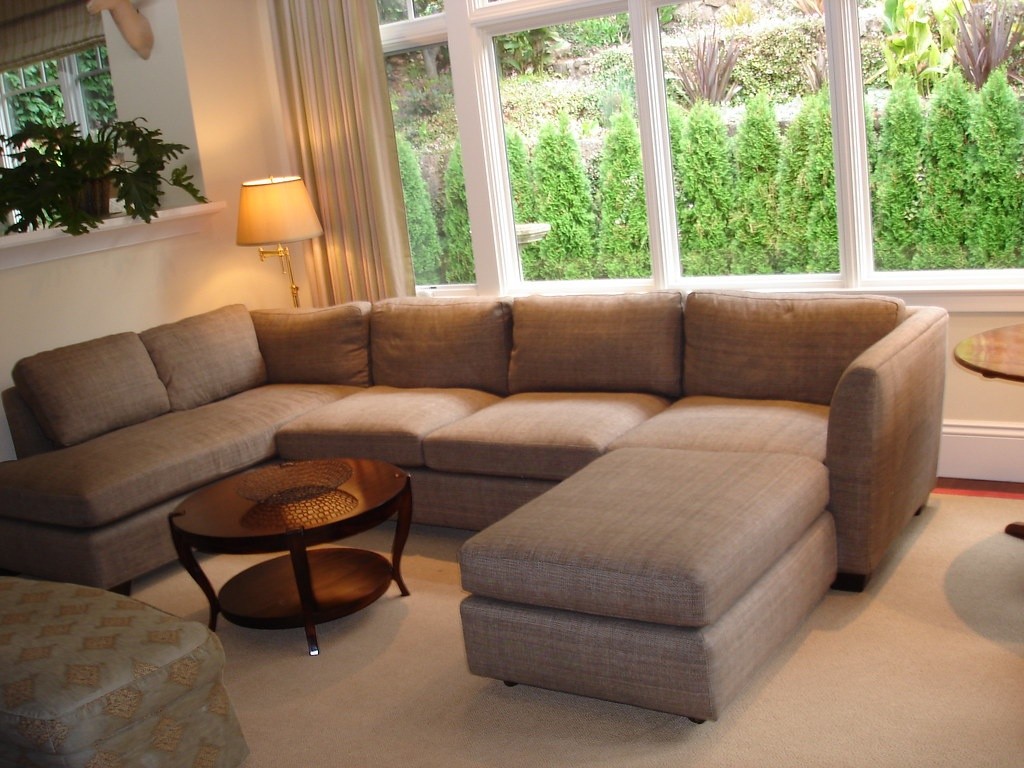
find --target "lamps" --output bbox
[234,173,325,310]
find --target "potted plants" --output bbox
[0,108,210,236]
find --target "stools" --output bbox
[0,573,251,768]
[457,447,838,725]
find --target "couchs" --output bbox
[0,291,952,598]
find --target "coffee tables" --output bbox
[168,457,413,657]
[954,321,1024,540]
